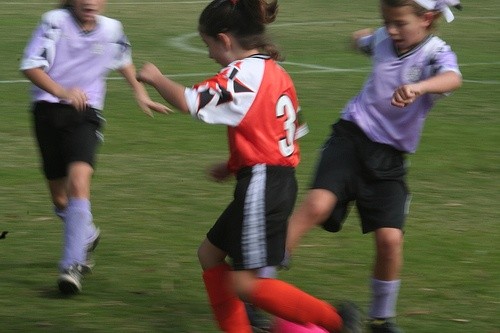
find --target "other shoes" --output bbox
[79,235,99,271]
[338,301,362,333]
[370,320,396,333]
[59,269,81,297]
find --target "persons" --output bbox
[271,0,465,333]
[17,1,176,297]
[134,0,362,333]
